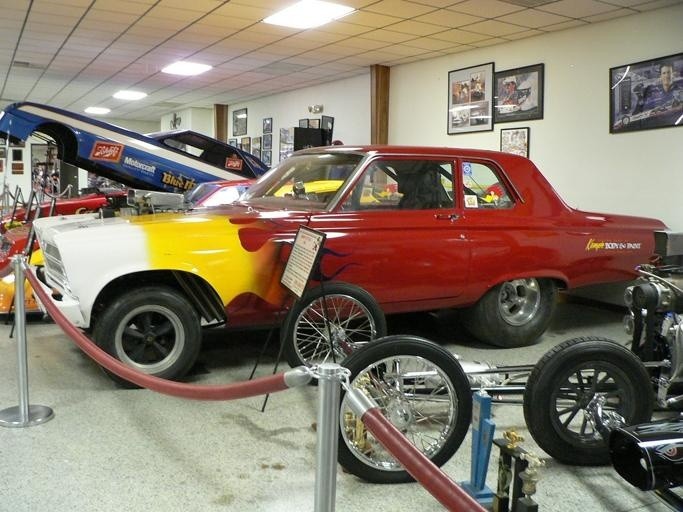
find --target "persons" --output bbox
[645,59,682,126]
[451,72,520,126]
[325,139,357,182]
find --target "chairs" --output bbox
[394,166,443,209]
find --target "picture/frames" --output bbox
[499,126,531,159]
[278,224,327,303]
[225,107,334,167]
[446,62,494,136]
[609,52,683,135]
[493,63,545,124]
